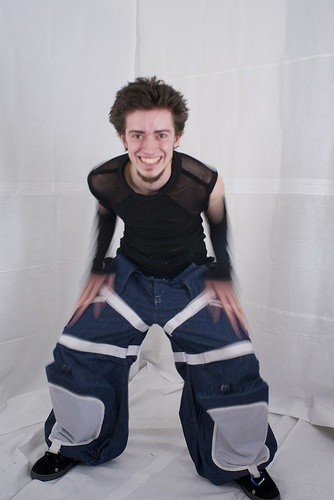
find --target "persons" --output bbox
[28,76,280,500]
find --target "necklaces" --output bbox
[136,167,167,193]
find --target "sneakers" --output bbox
[30,451,75,481]
[236,468,285,498]
